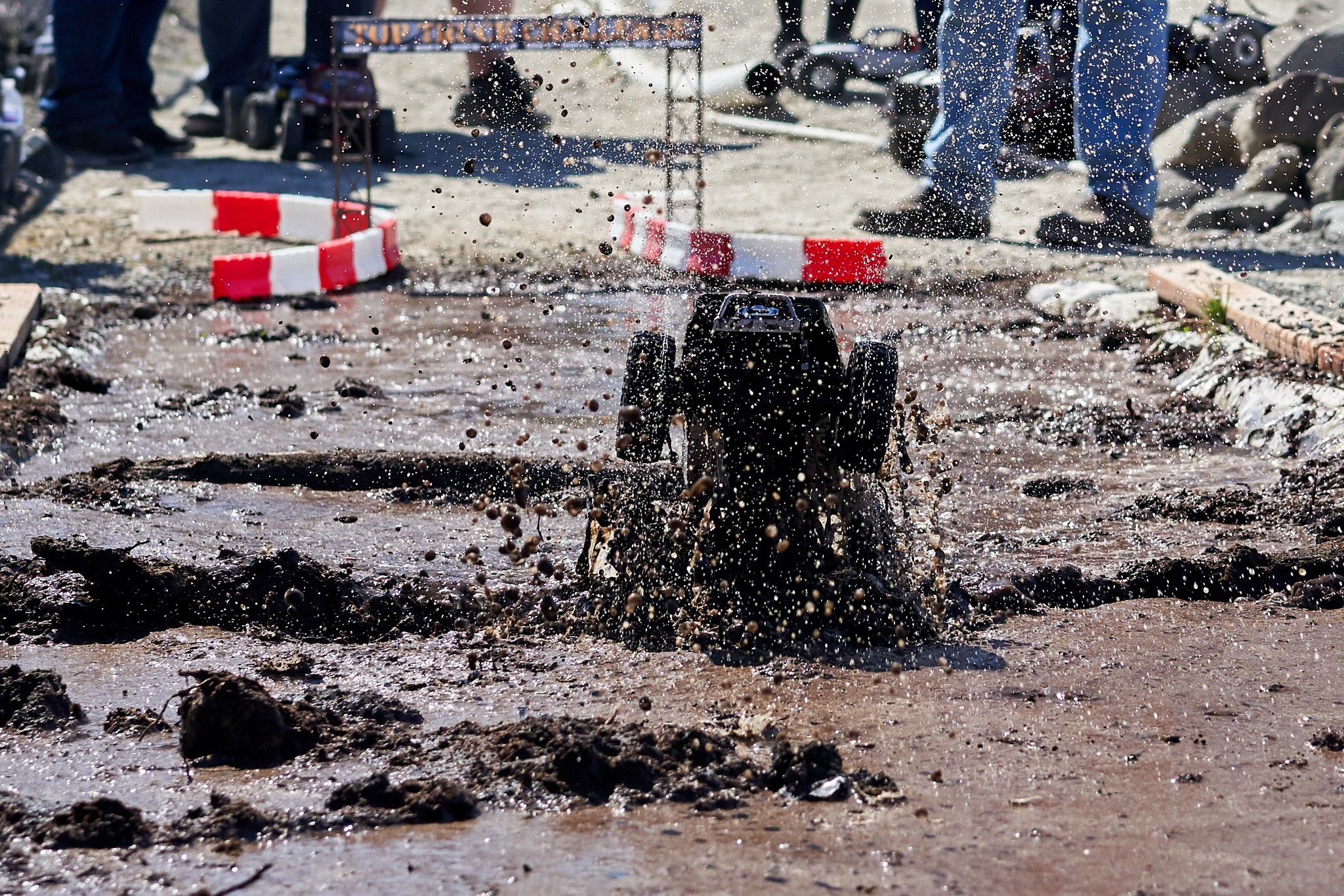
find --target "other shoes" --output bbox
[863,187,991,238]
[40,124,160,169]
[120,114,195,153]
[453,76,529,124]
[1037,199,1153,246]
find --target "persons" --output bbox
[372,0,552,127]
[38,0,197,166]
[181,0,395,149]
[774,0,862,64]
[855,0,1169,250]
